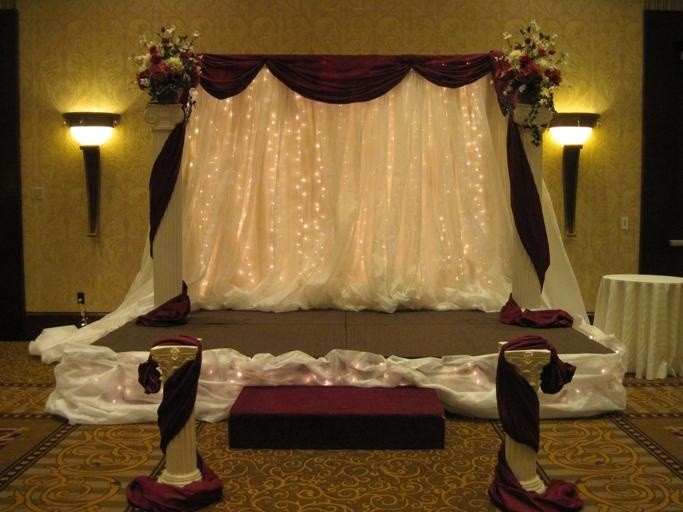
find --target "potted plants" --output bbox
[62,111,119,237]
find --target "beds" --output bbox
[90,306,624,421]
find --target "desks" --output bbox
[599,274,683,380]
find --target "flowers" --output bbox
[494,16,570,149]
[128,22,205,104]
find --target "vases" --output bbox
[142,104,184,131]
[512,104,552,127]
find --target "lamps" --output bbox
[550,111,602,238]
